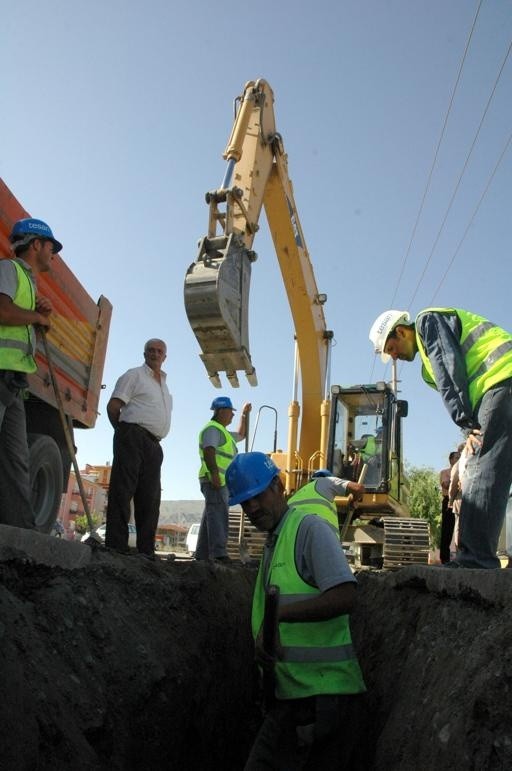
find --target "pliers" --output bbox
[237,403,252,564]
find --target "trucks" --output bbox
[0,176,116,535]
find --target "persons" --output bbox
[107,339,172,562]
[194,397,253,563]
[0,219,62,532]
[225,451,367,771]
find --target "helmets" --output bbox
[210,397,237,411]
[311,469,335,481]
[369,310,410,364]
[9,218,63,254]
[225,452,281,506]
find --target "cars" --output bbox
[80,522,157,549]
[50,518,66,540]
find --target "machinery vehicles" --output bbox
[184,77,431,565]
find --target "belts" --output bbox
[133,423,160,443]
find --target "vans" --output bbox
[185,521,201,558]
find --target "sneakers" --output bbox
[444,561,483,569]
[214,555,233,567]
